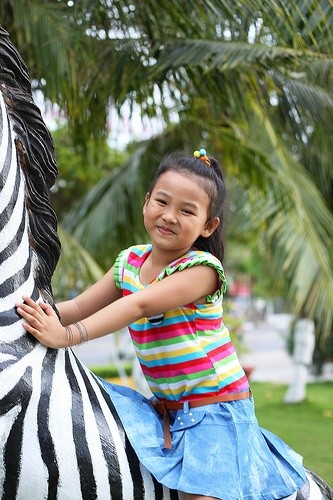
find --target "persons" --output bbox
[15,147,333,500]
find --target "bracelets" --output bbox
[64,321,89,348]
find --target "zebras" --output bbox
[0,23,333,500]
